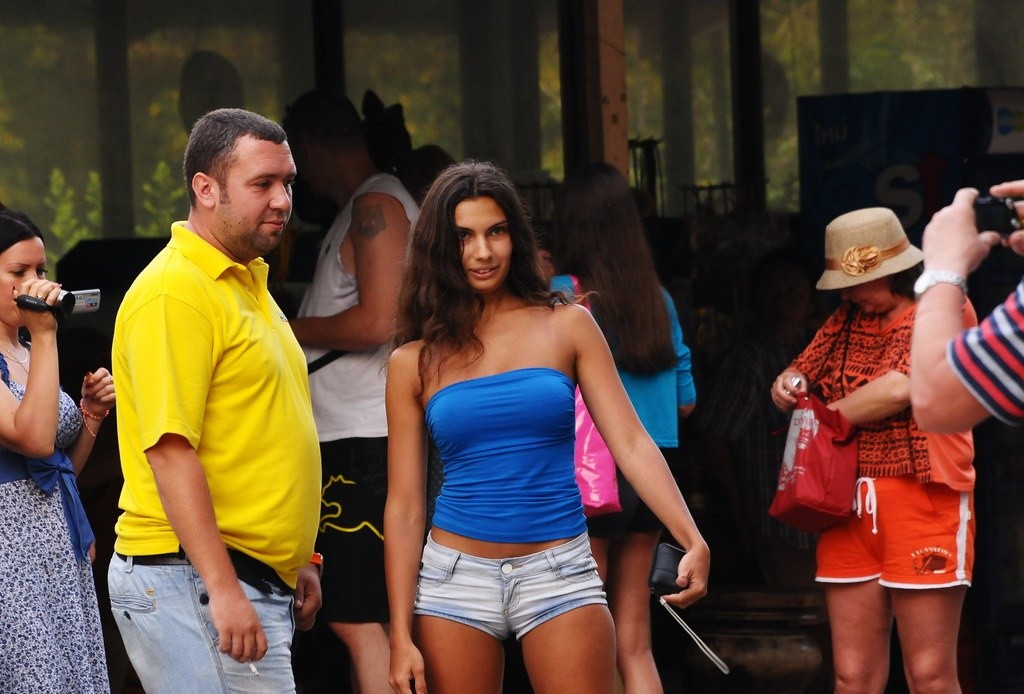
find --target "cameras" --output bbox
[56,288,100,317]
[787,377,802,394]
[972,197,1022,238]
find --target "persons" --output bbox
[539,161,698,694]
[770,205,977,694]
[383,161,709,693]
[909,179,1024,435]
[0,207,116,694]
[696,241,827,591]
[281,86,420,694]
[108,107,324,694]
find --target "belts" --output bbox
[112,539,288,595]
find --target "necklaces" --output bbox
[0,344,29,376]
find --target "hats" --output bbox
[814,207,925,291]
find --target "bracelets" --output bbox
[80,399,109,437]
[914,269,966,313]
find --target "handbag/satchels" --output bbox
[768,391,856,536]
[572,272,623,516]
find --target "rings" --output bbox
[37,295,45,300]
